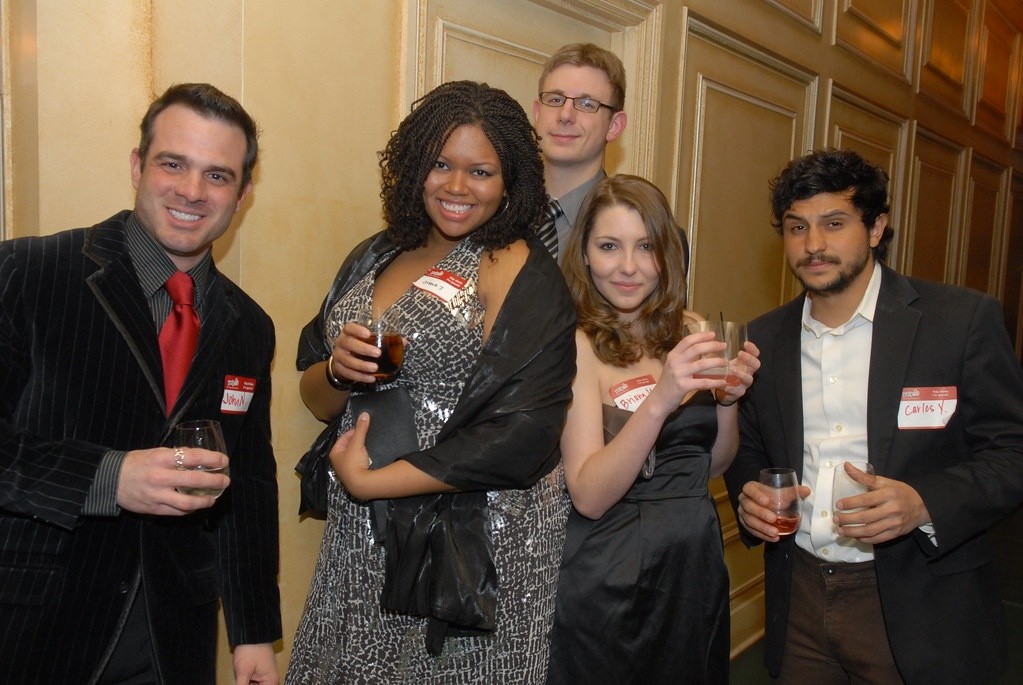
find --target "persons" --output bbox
[0,83,280,685]
[286,43,760,684]
[721,146,1023,684]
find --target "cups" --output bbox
[359,308,405,378]
[832,462,877,529]
[175,419,228,499]
[681,322,730,380]
[720,323,748,385]
[759,468,801,536]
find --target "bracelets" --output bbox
[325,355,352,391]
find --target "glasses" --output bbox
[539,90,621,113]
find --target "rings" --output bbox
[174,446,184,470]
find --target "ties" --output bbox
[534,198,564,263]
[158,271,202,418]
